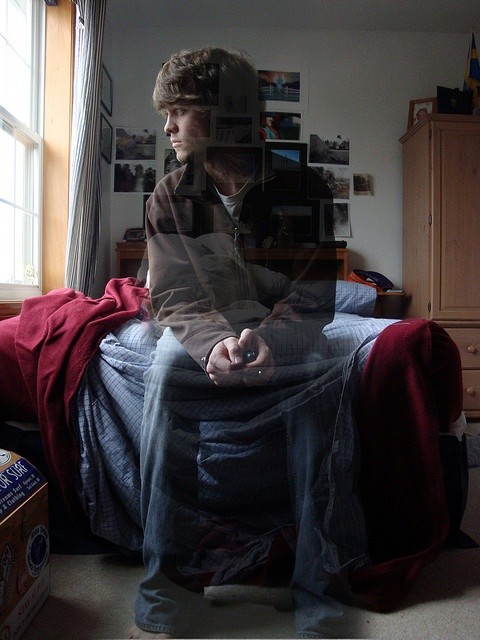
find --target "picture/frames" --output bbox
[100,62,112,116]
[407,96,438,131]
[99,112,113,165]
[266,142,307,164]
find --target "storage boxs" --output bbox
[0,448,52,640]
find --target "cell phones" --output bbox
[240,347,258,363]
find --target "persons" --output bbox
[127,47,349,639]
[334,135,346,150]
[262,115,279,138]
[416,108,427,120]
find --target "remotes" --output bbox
[387,288,403,293]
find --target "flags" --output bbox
[463,34,480,109]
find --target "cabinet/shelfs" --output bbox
[398,113,480,418]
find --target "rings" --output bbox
[259,370,264,377]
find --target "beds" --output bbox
[1,240,460,613]
